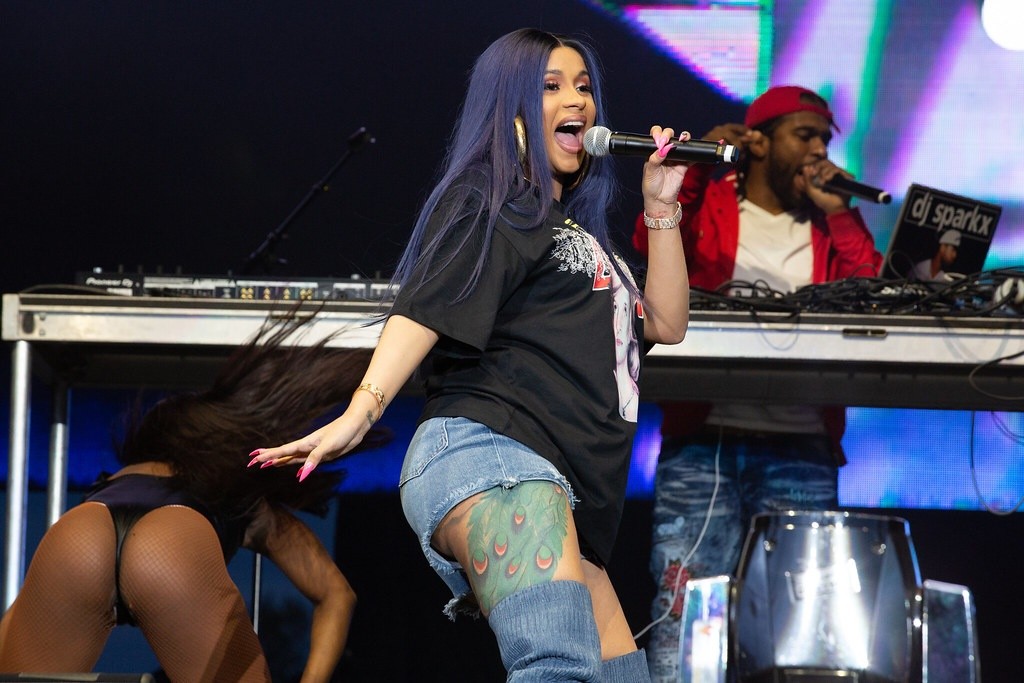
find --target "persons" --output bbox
[633,85,885,682]
[0,349,357,683]
[909,229,961,282]
[246,28,690,682]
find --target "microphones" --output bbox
[582,125,739,163]
[812,173,892,204]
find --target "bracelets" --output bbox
[644,201,681,231]
[352,383,386,423]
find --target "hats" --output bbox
[745,86,840,133]
[939,230,961,246]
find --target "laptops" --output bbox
[879,183,1002,282]
